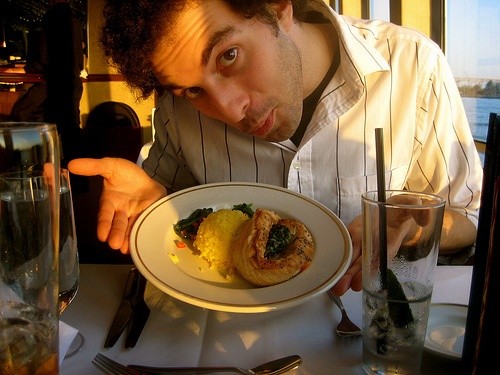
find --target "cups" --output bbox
[0,121,60,375]
[361,190,446,375]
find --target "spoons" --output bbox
[328,290,363,338]
[128,355,303,375]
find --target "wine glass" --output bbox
[0,167,85,360]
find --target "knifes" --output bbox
[103,267,151,349]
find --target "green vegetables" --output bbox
[264,224,291,259]
[174,203,255,236]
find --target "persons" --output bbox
[67,0,482,296]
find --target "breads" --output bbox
[229,208,313,286]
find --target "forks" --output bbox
[92,353,153,375]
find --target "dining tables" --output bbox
[52,263,473,375]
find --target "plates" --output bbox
[128,182,354,314]
[423,303,468,360]
